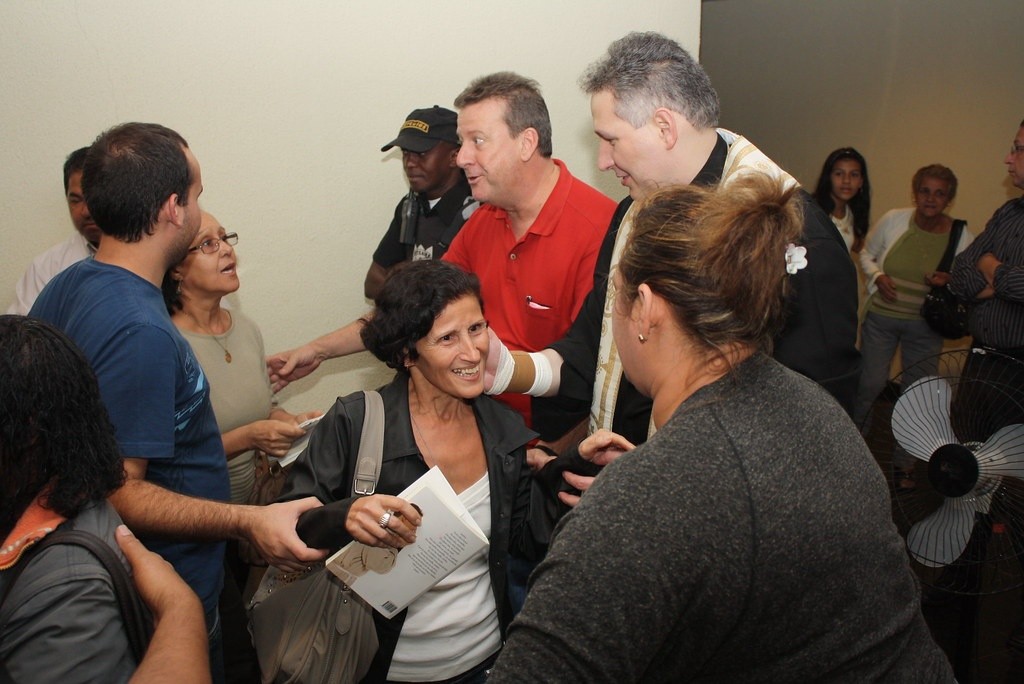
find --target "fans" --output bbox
[864,344,1023,671]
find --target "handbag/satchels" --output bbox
[245,390,385,684]
[237,446,292,567]
[921,219,970,340]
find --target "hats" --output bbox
[381,105,460,153]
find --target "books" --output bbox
[325,465,490,618]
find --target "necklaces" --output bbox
[177,303,232,364]
[410,415,437,466]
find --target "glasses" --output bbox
[1010,145,1024,154]
[186,232,238,255]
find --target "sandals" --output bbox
[896,464,917,492]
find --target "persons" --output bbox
[162,210,324,593]
[807,148,871,258]
[363,105,485,301]
[0,316,152,684]
[936,116,1024,672]
[266,71,619,431]
[266,259,636,683]
[327,502,424,586]
[114,523,214,684]
[6,148,96,316]
[484,31,859,450]
[25,121,325,684]
[488,174,958,684]
[852,163,978,493]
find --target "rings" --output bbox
[379,512,392,527]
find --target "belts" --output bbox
[972,343,1024,360]
